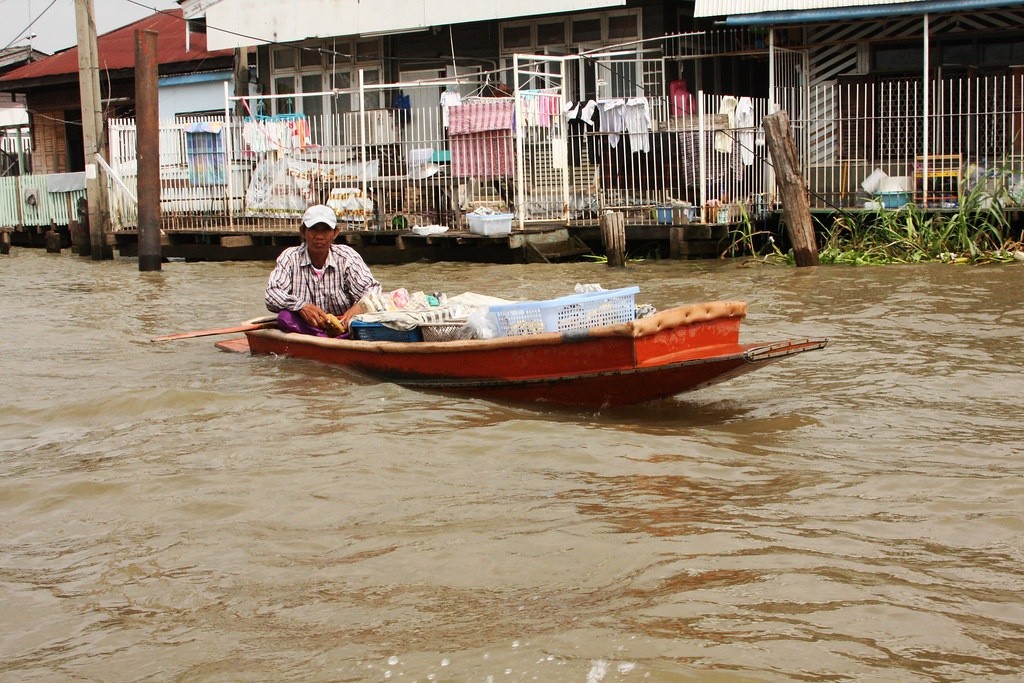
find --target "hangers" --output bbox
[460,70,514,97]
[518,61,561,90]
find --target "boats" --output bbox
[214,300,829,410]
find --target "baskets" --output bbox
[487,284,642,337]
[417,320,475,342]
[350,320,423,343]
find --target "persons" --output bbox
[264,204,382,340]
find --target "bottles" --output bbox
[717,209,729,225]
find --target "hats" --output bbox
[301,205,337,229]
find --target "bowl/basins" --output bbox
[654,205,697,222]
[873,191,913,208]
[428,150,450,162]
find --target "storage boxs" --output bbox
[656,206,697,223]
[350,286,643,341]
[465,212,514,235]
[873,190,912,207]
